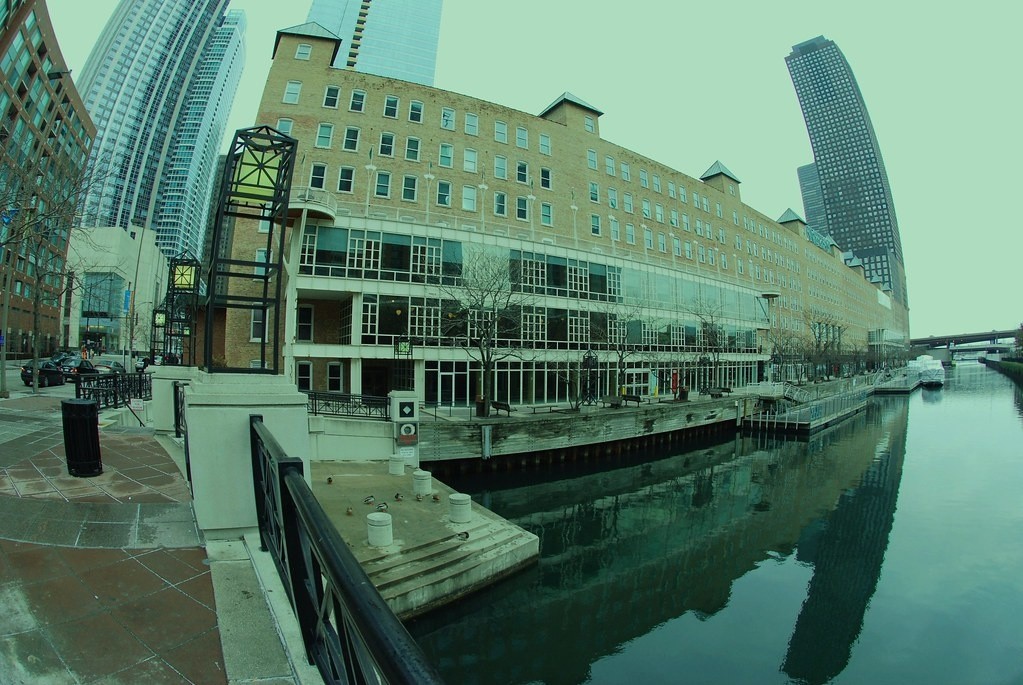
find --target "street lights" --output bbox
[128,219,146,373]
[0,67,73,398]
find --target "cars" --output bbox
[93,360,127,379]
[154,352,179,365]
[21,359,65,387]
[50,350,93,366]
[134,357,151,372]
[54,358,99,382]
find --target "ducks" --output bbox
[347,507,353,516]
[377,502,388,512]
[416,494,423,501]
[395,492,404,503]
[364,496,375,506]
[432,495,441,503]
[458,532,469,540]
[327,477,332,484]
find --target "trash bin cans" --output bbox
[680,386,689,401]
[475,395,486,417]
[60,399,104,479]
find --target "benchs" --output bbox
[527,404,559,414]
[717,388,733,397]
[622,394,645,407]
[814,377,824,383]
[490,400,518,417]
[644,397,665,404]
[785,381,794,386]
[597,396,623,409]
[709,389,724,399]
